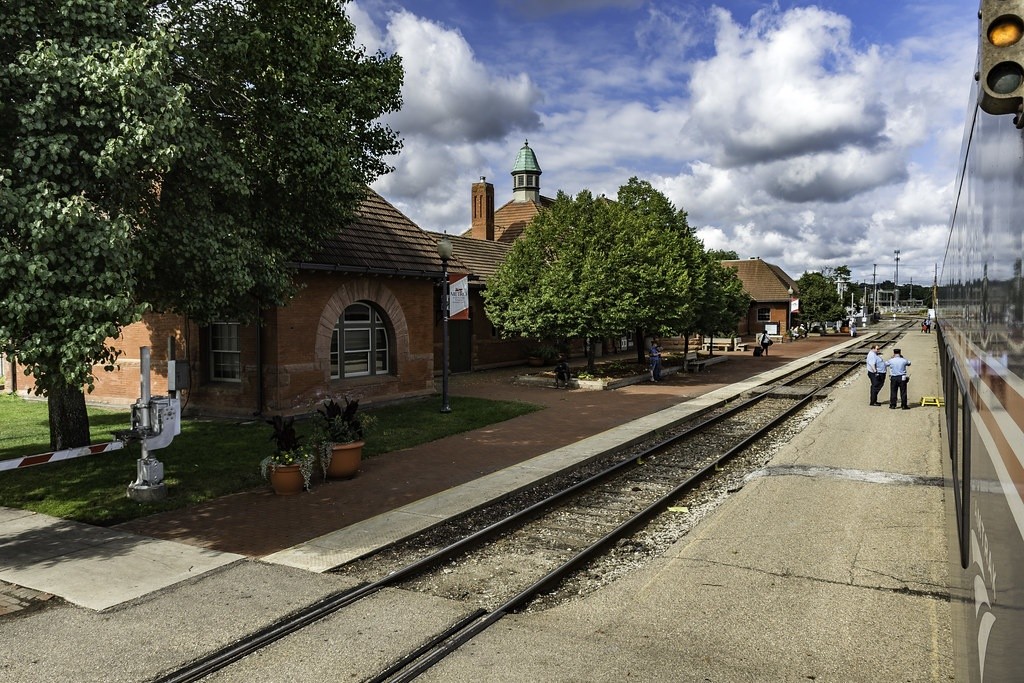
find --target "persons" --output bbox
[758,330,771,357]
[790,324,808,339]
[921,319,931,333]
[866,344,887,406]
[884,348,912,409]
[648,340,664,383]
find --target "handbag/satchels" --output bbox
[769,340,773,346]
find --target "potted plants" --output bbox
[259,397,365,494]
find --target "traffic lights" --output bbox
[975,0,1023,111]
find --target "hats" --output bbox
[893,349,901,354]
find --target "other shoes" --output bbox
[870,402,881,406]
[889,405,895,409]
[902,405,910,409]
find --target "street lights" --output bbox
[787,284,795,343]
[436,229,452,414]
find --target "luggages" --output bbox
[753,346,762,357]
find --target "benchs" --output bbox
[703,338,749,352]
